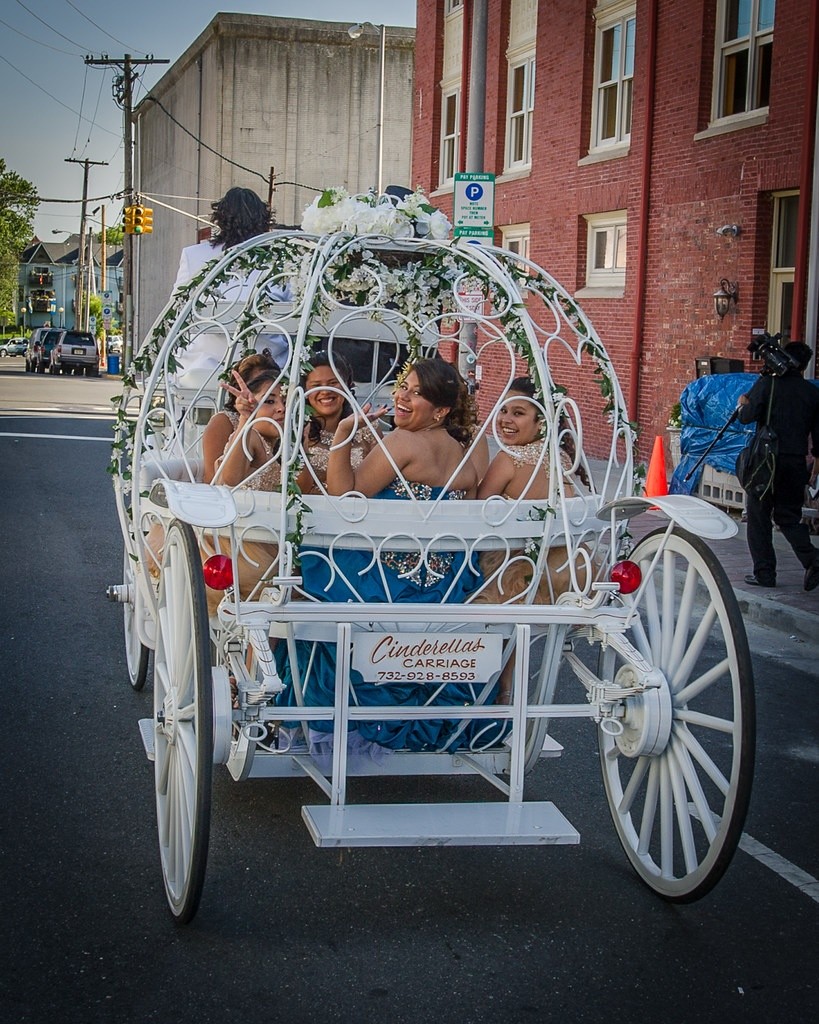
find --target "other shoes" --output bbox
[257,726,279,749]
[494,690,510,704]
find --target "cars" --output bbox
[0,337,30,357]
[106,336,123,353]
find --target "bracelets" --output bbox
[737,404,744,412]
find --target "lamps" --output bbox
[714,279,739,322]
[717,225,741,237]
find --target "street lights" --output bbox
[59,306,64,329]
[21,307,27,337]
[349,23,387,201]
[52,227,92,332]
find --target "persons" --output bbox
[448,363,489,482]
[737,341,819,591]
[202,369,301,706]
[294,350,383,494]
[469,376,596,704]
[170,187,294,388]
[268,359,513,764]
[143,354,281,580]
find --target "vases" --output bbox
[665,426,682,470]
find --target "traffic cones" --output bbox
[642,433,673,510]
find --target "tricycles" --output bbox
[111,186,755,926]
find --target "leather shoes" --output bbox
[804,555,819,592]
[745,575,776,587]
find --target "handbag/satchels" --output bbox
[736,426,780,501]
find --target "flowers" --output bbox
[668,403,684,428]
[106,185,648,587]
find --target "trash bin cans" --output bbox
[108,354,119,374]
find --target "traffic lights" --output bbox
[122,206,153,235]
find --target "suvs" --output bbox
[26,329,99,378]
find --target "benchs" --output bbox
[142,304,640,643]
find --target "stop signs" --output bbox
[454,174,493,245]
